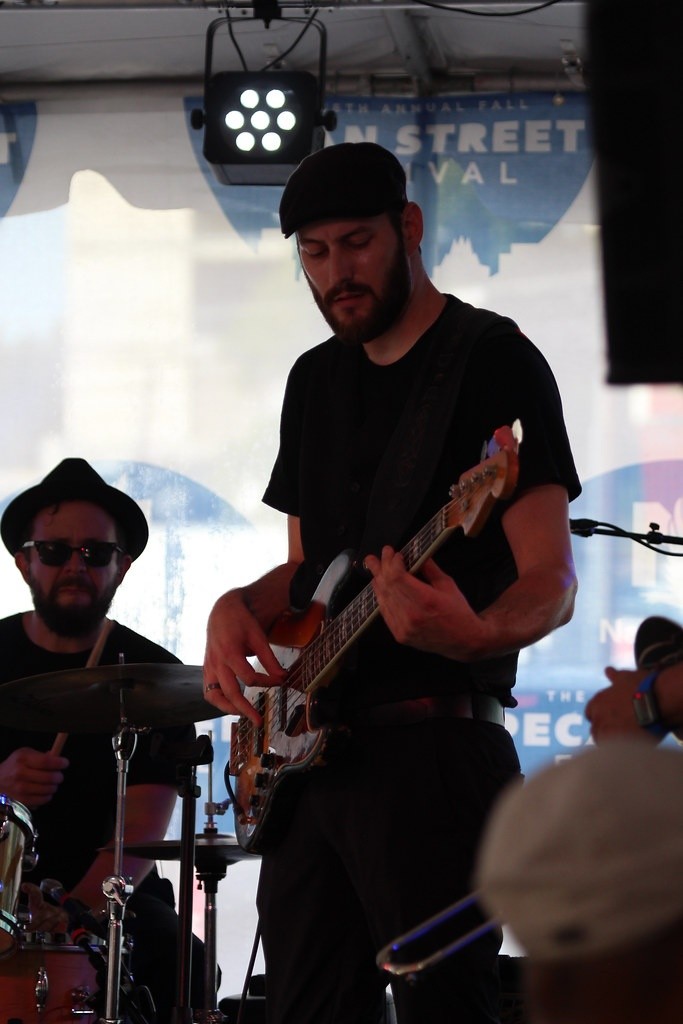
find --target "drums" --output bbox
[0,943,130,1024]
[0,793,38,960]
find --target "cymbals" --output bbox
[0,664,246,738]
[96,832,262,875]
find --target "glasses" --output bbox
[21,540,126,566]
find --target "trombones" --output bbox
[376,882,511,977]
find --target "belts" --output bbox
[325,690,504,728]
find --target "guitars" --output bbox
[227,417,524,854]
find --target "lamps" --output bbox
[188,10,340,187]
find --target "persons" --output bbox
[204,141,580,1023]
[586,659,683,754]
[377,740,683,1023]
[0,457,220,1024]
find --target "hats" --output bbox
[1,458,149,563]
[280,143,406,239]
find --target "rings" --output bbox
[206,684,220,693]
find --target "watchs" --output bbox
[632,671,668,736]
